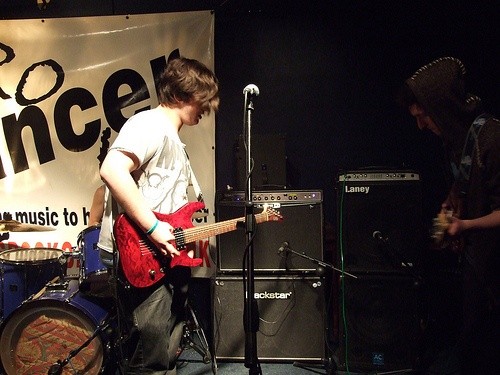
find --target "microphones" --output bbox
[243,83,260,98]
[373,230,385,242]
[277,241,290,255]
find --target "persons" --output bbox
[85,158,126,276]
[396,54,500,356]
[98,54,218,375]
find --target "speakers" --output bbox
[212,274,326,362]
[336,178,424,274]
[216,189,325,273]
[336,274,419,375]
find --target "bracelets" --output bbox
[145,219,160,237]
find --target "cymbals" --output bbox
[0,220,56,232]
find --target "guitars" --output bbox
[114,201,285,288]
[431,182,465,257]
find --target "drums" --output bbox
[76,223,118,299]
[0,277,119,375]
[0,248,67,324]
[66,251,80,276]
